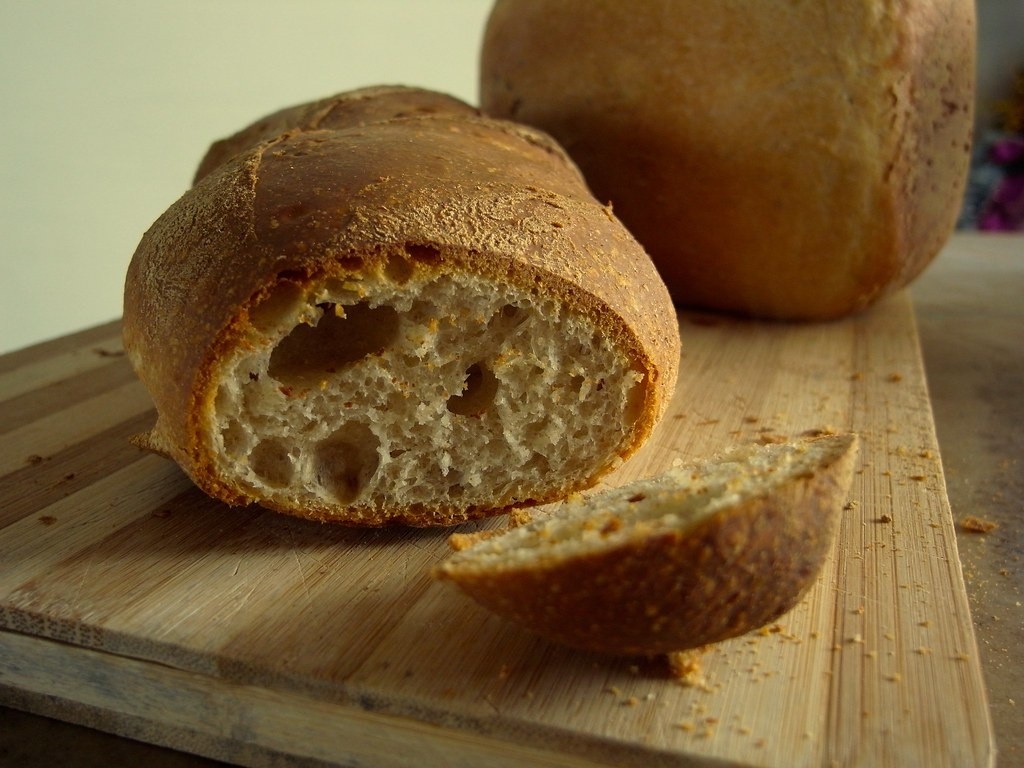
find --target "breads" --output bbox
[479,1,977,314]
[437,423,858,651]
[125,86,680,523]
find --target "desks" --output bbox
[1,229,1024,768]
